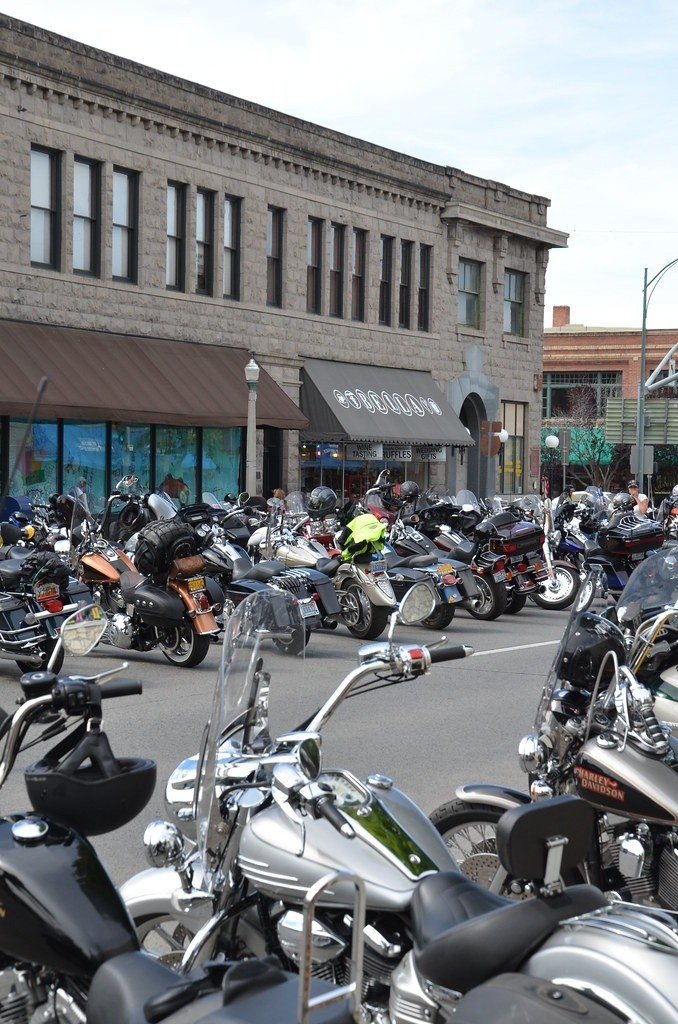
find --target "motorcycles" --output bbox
[0,544,678,1024]
[0,475,677,678]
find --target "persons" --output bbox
[628,480,649,520]
[267,488,288,515]
[344,494,357,512]
[556,485,578,509]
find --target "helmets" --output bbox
[474,522,498,543]
[10,511,29,528]
[23,756,157,837]
[224,493,237,503]
[117,503,147,531]
[580,522,602,534]
[556,612,626,691]
[613,493,637,509]
[307,486,337,516]
[401,481,419,498]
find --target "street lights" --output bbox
[244,351,260,498]
[544,434,560,500]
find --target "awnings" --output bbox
[0,318,310,432]
[299,357,476,447]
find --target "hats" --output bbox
[627,480,640,488]
